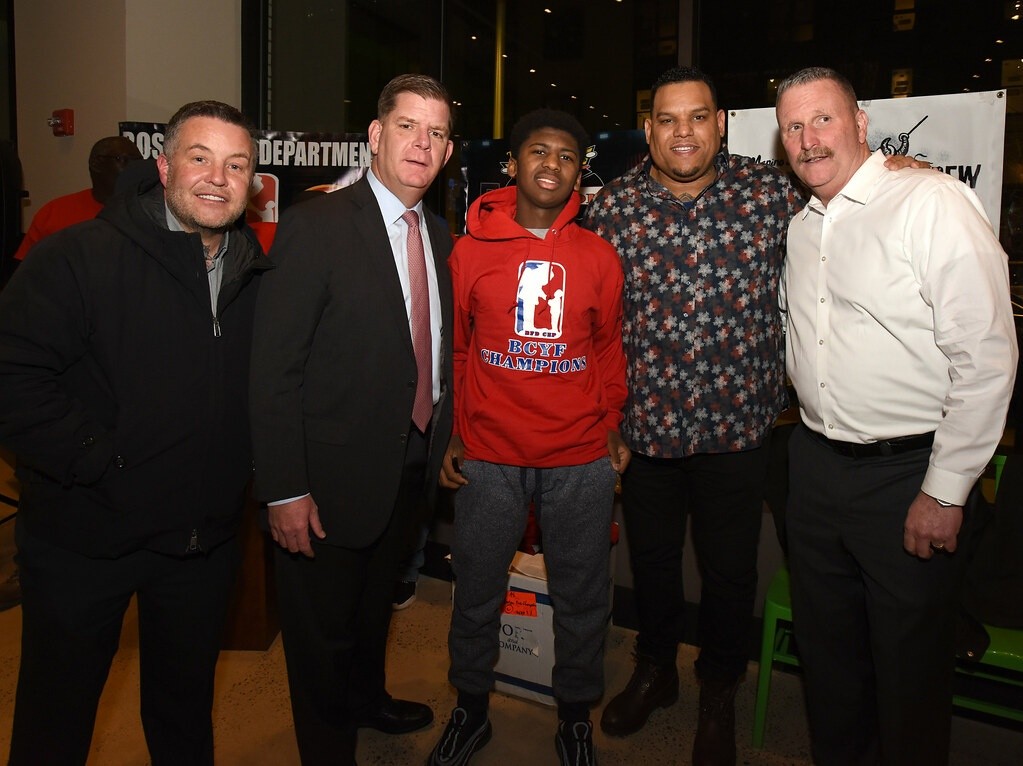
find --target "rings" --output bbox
[931,543,945,552]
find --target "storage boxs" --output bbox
[451,540,618,706]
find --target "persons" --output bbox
[580,66,933,766]
[422,107,631,766]
[775,66,1020,766]
[1,74,462,766]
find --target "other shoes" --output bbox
[391,581,418,610]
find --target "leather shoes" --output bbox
[358,697,433,734]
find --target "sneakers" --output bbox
[600,645,680,736]
[693,680,737,766]
[427,704,492,766]
[555,719,598,766]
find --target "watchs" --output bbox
[936,500,951,507]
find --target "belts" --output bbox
[817,433,934,457]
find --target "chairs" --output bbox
[747,449,1022,750]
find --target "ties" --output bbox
[401,210,434,434]
